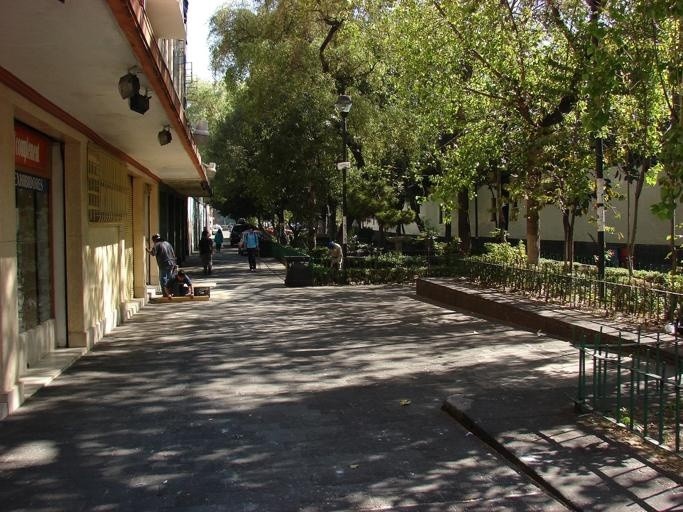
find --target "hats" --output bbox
[152,233,160,240]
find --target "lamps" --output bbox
[118,73,172,147]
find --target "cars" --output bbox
[230,223,256,246]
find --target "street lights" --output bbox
[334,93,352,284]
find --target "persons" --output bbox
[215,229,223,251]
[244,228,258,272]
[146,234,175,298]
[327,242,343,270]
[165,270,194,299]
[199,231,213,274]
